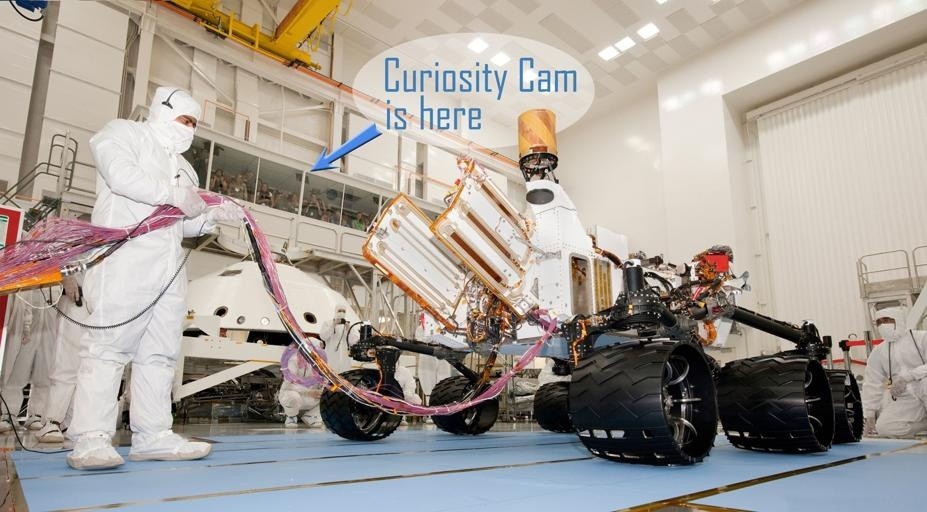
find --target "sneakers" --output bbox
[129,429,210,461]
[66,437,125,470]
[0,413,64,445]
[285,410,322,428]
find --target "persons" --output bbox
[278,336,323,429]
[321,304,358,373]
[66,86,244,469]
[210,168,366,232]
[0,213,91,443]
[861,307,927,439]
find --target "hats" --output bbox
[153,85,201,126]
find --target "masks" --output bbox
[173,123,195,152]
[878,323,894,338]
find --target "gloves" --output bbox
[171,184,245,222]
[889,378,907,395]
[863,417,878,437]
